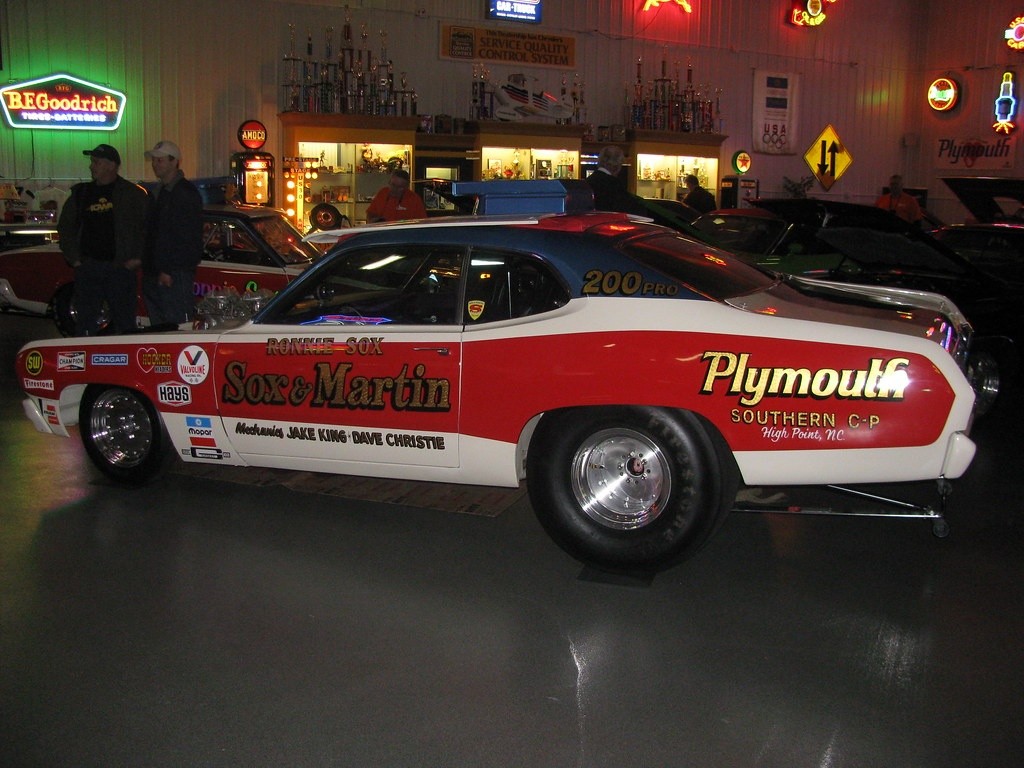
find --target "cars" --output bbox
[0,202,406,337]
[29,205,981,576]
[595,193,1024,396]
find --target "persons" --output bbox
[874,175,922,227]
[143,141,203,325]
[684,176,716,213]
[586,145,625,211]
[366,170,427,224]
[57,144,148,335]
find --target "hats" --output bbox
[82,144,122,167]
[144,141,181,163]
